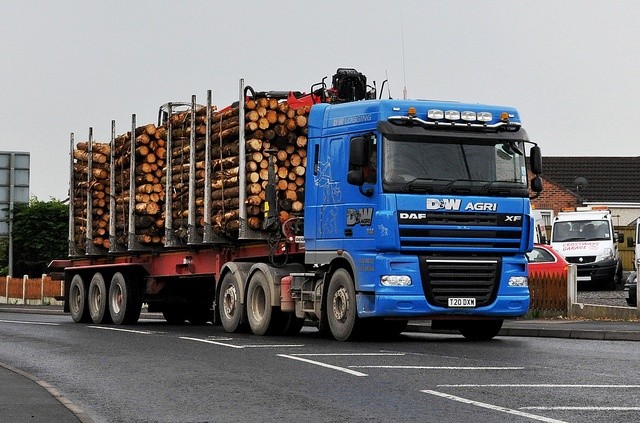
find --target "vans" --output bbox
[529,209,547,244]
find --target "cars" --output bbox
[525,243,572,280]
[624,271,638,307]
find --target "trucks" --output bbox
[550,205,624,291]
[46,68,542,341]
